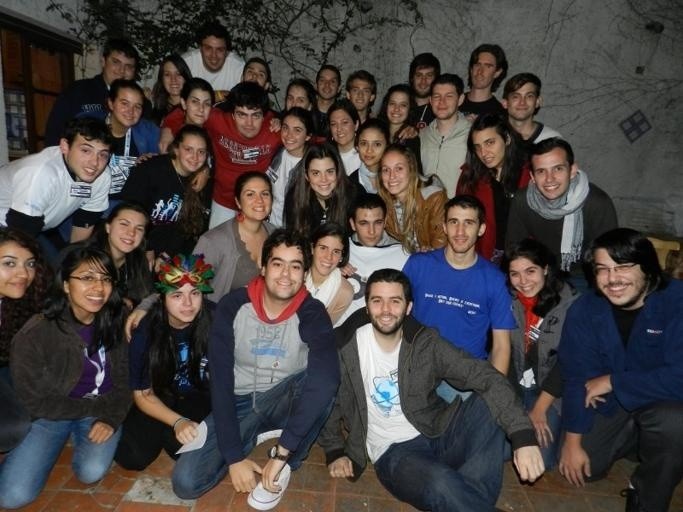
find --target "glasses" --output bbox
[593,263,639,276]
[69,274,113,284]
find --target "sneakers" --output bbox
[620,487,638,512]
[244,460,293,511]
